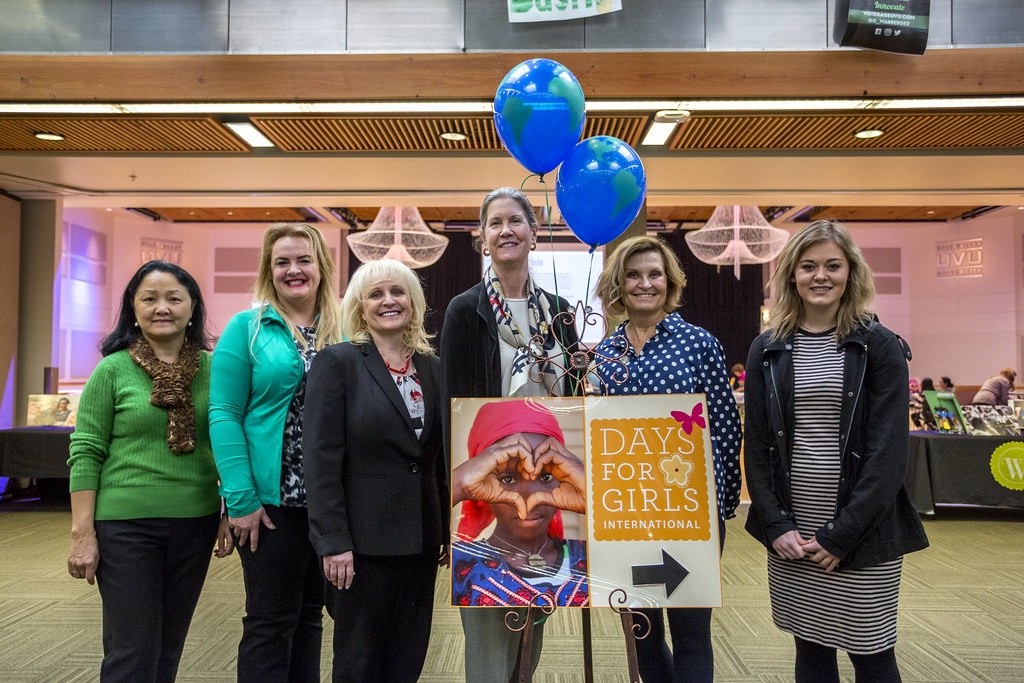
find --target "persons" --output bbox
[594,237,745,683]
[971,367,1017,406]
[911,405,1020,436]
[452,400,592,608]
[210,223,351,683]
[940,376,953,393]
[301,258,452,683]
[731,363,745,392]
[440,187,584,683]
[912,377,935,431]
[67,259,235,683]
[744,218,930,683]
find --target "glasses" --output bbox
[911,384,919,388]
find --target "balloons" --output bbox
[492,57,645,252]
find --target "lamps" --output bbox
[346,206,449,269]
[684,204,790,279]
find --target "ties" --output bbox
[484,265,562,397]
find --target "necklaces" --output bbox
[492,533,549,569]
[385,357,410,374]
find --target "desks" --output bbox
[0,424,71,506]
[905,431,1024,514]
